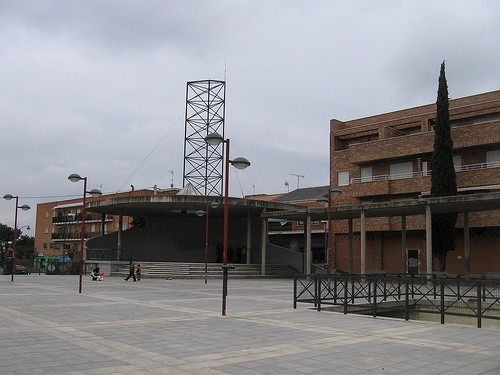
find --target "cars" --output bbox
[3,265,30,275]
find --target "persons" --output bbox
[124,260,138,281]
[91,267,100,281]
[136,265,141,281]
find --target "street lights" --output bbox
[204,134,251,314]
[316,188,342,273]
[3,194,31,281]
[12,225,32,264]
[68,174,102,294]
